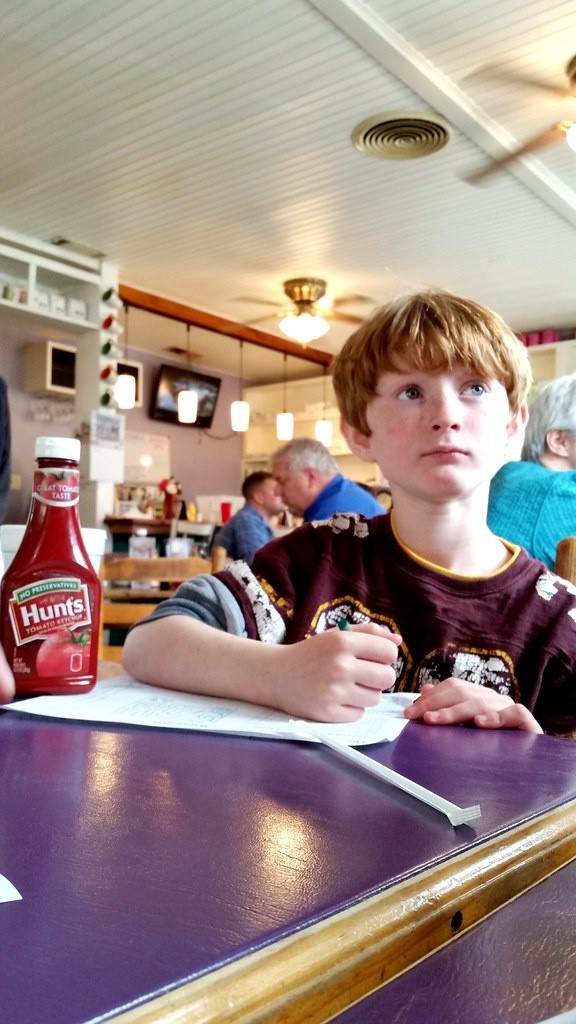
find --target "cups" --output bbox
[130,536,155,589]
[165,537,194,589]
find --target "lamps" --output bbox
[275,307,332,349]
[112,304,334,449]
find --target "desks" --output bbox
[0,700,576,1024]
[103,515,172,556]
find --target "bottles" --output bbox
[0,437,102,696]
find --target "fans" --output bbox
[231,276,376,329]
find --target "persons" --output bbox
[209,471,287,564]
[0,647,16,707]
[271,438,387,521]
[120,291,576,735]
[486,371,576,572]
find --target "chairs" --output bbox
[100,554,212,662]
